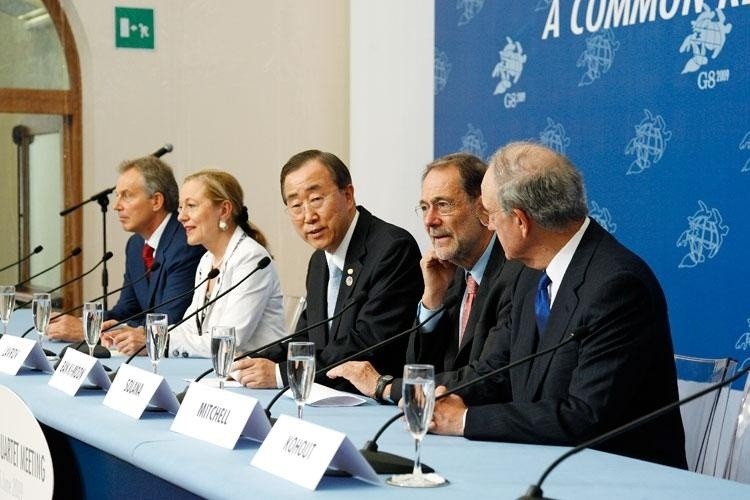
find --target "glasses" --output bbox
[285,190,346,212]
[414,198,479,217]
[478,206,507,226]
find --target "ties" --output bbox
[144,246,156,284]
[324,260,344,336]
[461,274,479,341]
[534,272,552,338]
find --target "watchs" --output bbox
[376,374,393,403]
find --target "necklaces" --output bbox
[212,256,223,268]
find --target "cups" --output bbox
[0,285,16,335]
[401,363,436,488]
[30,293,52,347]
[143,313,168,374]
[210,324,237,390]
[82,302,104,356]
[286,340,317,421]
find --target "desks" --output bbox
[0,307,750,500]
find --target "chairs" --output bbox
[719,363,750,486]
[675,356,735,471]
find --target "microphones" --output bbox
[322,327,592,478]
[144,292,372,412]
[12,251,114,311]
[18,262,163,358]
[516,366,750,499]
[1,245,44,273]
[150,142,175,163]
[9,246,81,290]
[60,266,220,358]
[263,293,464,426]
[81,256,272,391]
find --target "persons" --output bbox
[43,156,206,343]
[397,140,690,471]
[326,152,526,404]
[231,150,424,389]
[102,169,287,359]
